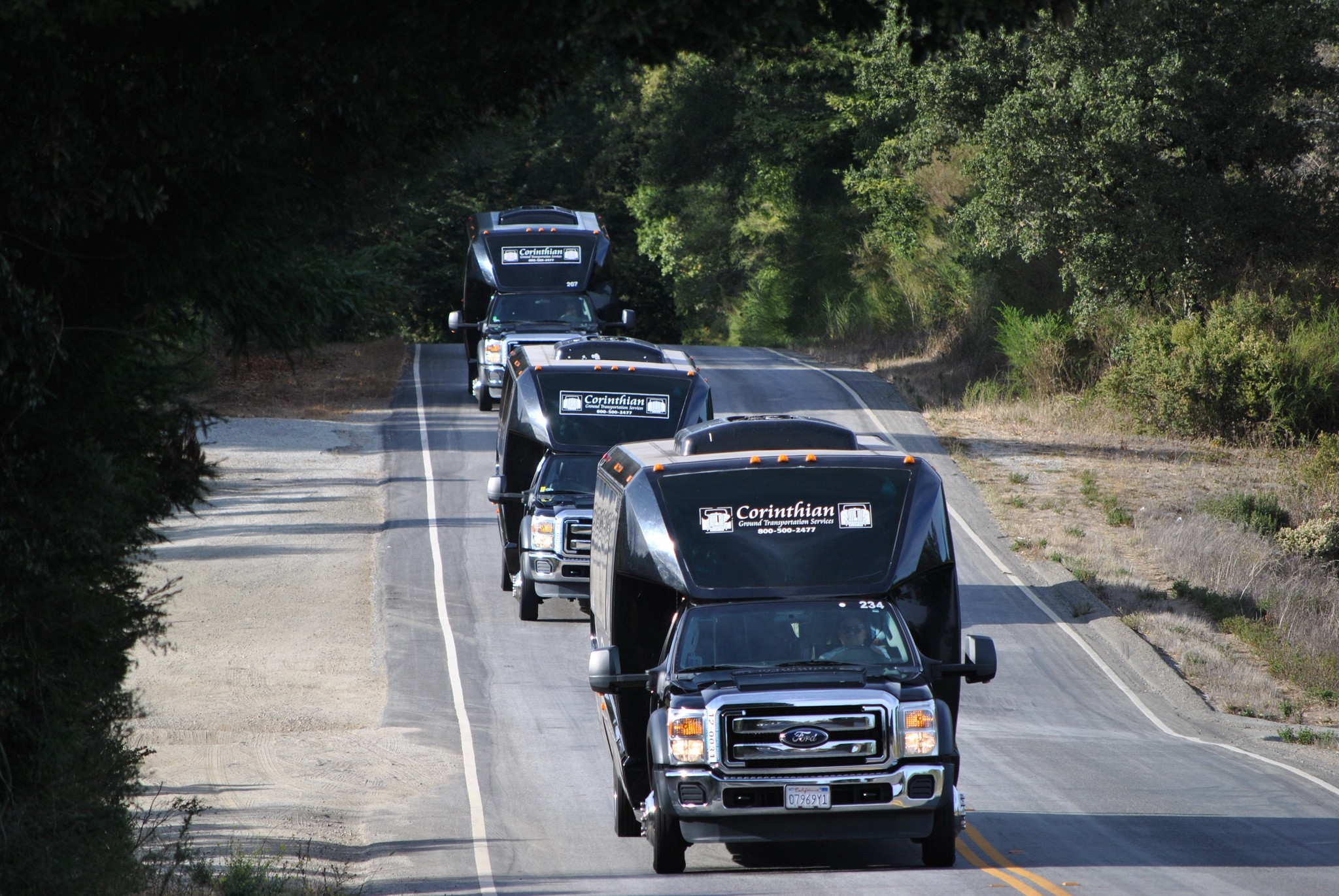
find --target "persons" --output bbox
[839,617,886,654]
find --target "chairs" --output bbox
[811,626,874,661]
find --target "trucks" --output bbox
[486,331,718,622]
[587,412,1000,876]
[448,203,638,413]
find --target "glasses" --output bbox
[843,624,864,634]
